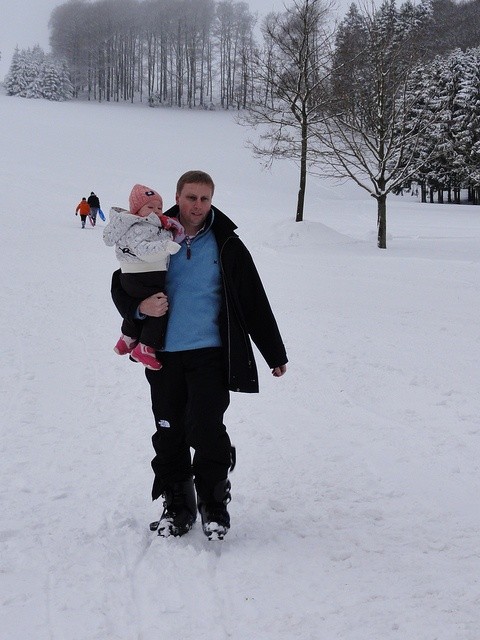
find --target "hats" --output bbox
[129,184,162,215]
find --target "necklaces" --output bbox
[178,220,206,258]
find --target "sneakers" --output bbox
[201,504,230,540]
[157,505,197,538]
[113,337,139,355]
[131,342,162,370]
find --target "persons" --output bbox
[88,192,100,226]
[112,170,288,540]
[75,198,90,228]
[104,184,182,372]
